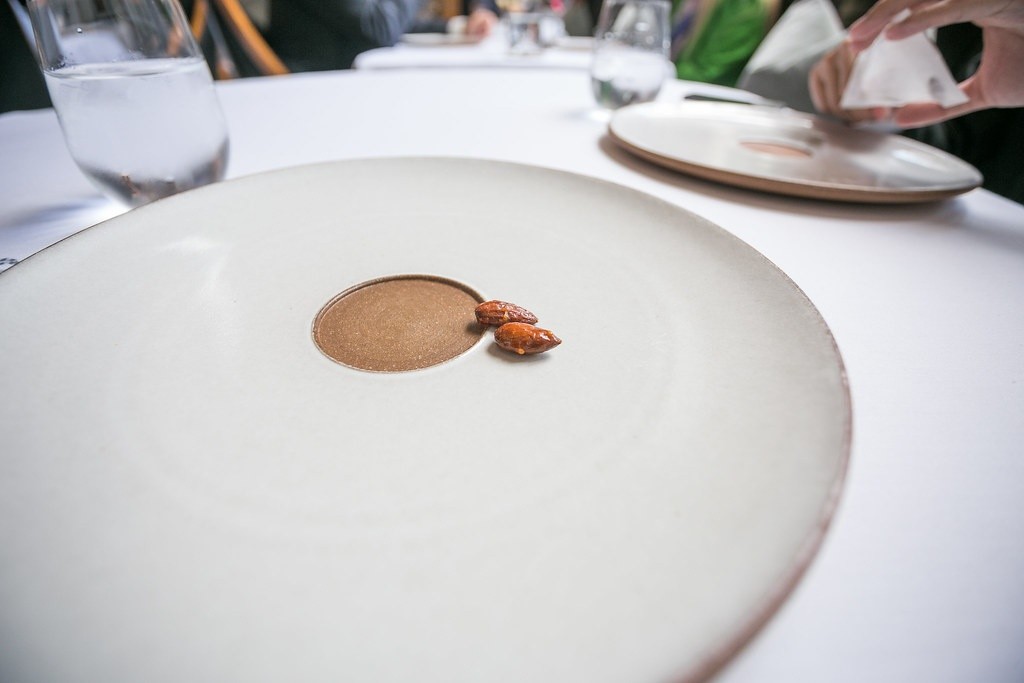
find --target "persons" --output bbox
[269,1,496,72]
[669,1,1024,212]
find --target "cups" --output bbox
[23,0,230,207]
[588,0,674,113]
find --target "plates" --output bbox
[1,157,853,681]
[608,100,985,202]
